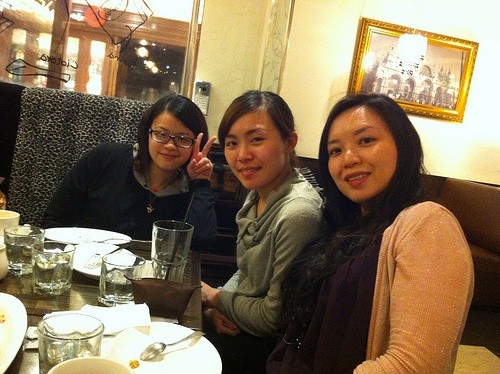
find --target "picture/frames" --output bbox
[348,16,482,126]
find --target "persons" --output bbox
[201,90,323,374]
[264,92,475,374]
[42,94,217,250]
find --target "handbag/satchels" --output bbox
[123,275,203,319]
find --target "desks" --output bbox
[0,236,203,374]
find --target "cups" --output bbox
[37,313,105,374]
[151,221,194,283]
[0,210,20,242]
[47,356,133,374]
[31,241,75,296]
[97,254,146,308]
[4,225,45,276]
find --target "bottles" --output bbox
[0,244,8,280]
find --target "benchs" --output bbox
[437,179,500,305]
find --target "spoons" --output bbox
[139,330,205,361]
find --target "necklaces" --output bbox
[147,175,171,213]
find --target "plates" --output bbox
[0,291,28,374]
[109,321,223,374]
[65,243,135,279]
[45,227,132,245]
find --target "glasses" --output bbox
[149,128,194,149]
